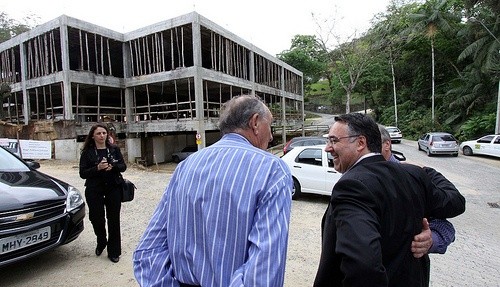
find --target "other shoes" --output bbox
[111,257,119,262]
[96,248,103,256]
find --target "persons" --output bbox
[79,124,127,263]
[132,94,292,287]
[313,113,466,287]
[376,121,456,258]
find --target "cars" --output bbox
[459,134,500,157]
[280,144,342,200]
[385,126,402,143]
[283,136,331,156]
[0,144,87,267]
[171,144,198,164]
[418,132,459,157]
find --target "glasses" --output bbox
[326,135,368,145]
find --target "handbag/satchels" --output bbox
[121,179,137,202]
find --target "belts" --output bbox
[177,280,201,287]
